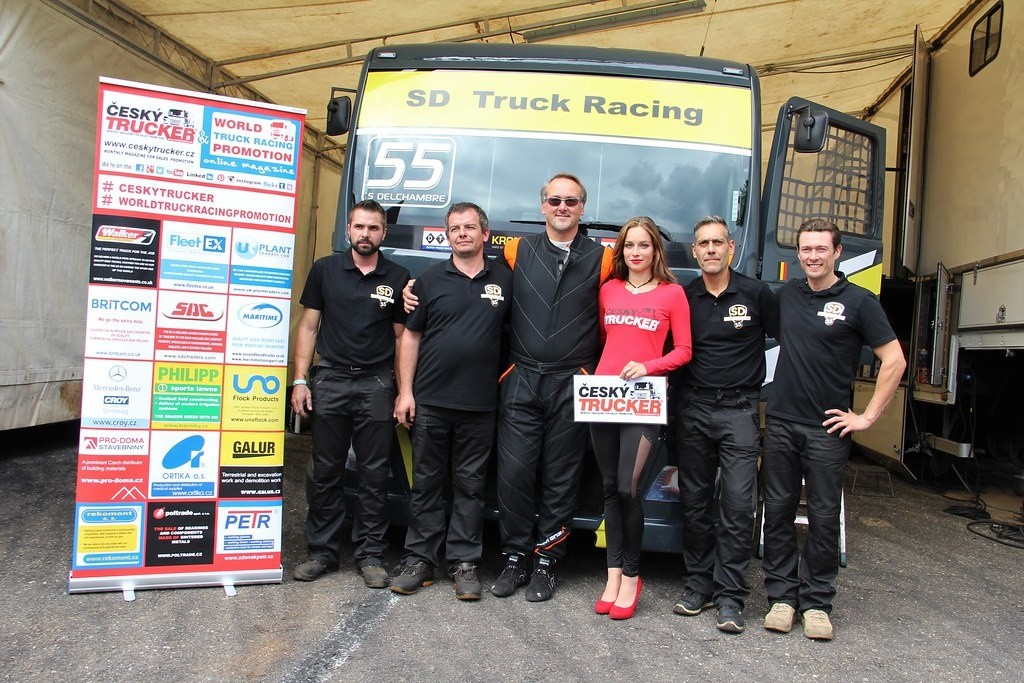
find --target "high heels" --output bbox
[594,586,618,614]
[610,575,642,619]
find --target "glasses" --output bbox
[546,197,580,207]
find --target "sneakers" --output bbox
[672,589,714,615]
[717,605,744,632]
[491,556,534,598]
[800,609,834,640]
[293,559,328,581]
[390,560,436,594]
[763,602,799,632]
[448,562,481,599]
[361,564,389,588]
[529,554,558,601]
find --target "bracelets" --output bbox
[293,380,306,386]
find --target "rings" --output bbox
[626,367,631,373]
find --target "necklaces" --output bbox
[628,277,653,295]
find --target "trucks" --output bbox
[327,44,886,555]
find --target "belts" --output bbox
[309,360,363,379]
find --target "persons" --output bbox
[390,202,516,601]
[761,219,906,639]
[667,214,781,632]
[291,200,410,588]
[589,216,692,619]
[402,173,616,601]
